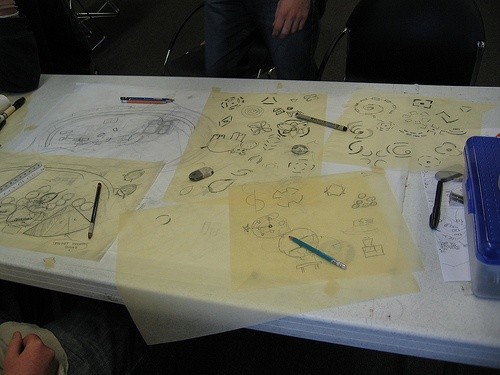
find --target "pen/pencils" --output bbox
[0,97,26,130]
[428,180,444,230]
[289,235,348,270]
[294,112,348,133]
[86,183,103,240]
[118,95,175,105]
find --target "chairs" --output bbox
[164,1,320,79]
[316,1,489,85]
[69,0,120,57]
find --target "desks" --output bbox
[0,74,500,371]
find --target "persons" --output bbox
[201,1,330,79]
[0,280,161,375]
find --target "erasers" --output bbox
[187,166,215,182]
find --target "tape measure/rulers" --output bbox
[1,162,47,203]
